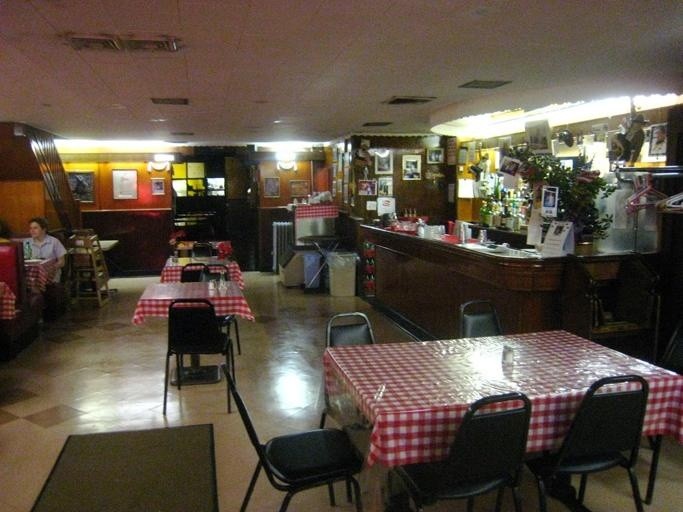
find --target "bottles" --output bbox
[478,183,531,231]
[401,207,418,218]
[24,240,32,260]
[359,240,377,295]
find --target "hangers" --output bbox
[625,173,683,214]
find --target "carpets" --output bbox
[30,424,219,512]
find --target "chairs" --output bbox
[161,299,237,416]
[222,298,650,512]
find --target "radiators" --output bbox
[272,221,295,274]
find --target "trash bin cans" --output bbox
[327,252,357,296]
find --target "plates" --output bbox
[24,259,42,265]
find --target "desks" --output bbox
[303,235,354,291]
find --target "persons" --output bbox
[608,96,648,167]
[21,217,66,324]
[649,125,668,155]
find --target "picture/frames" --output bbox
[151,178,166,196]
[263,177,311,199]
